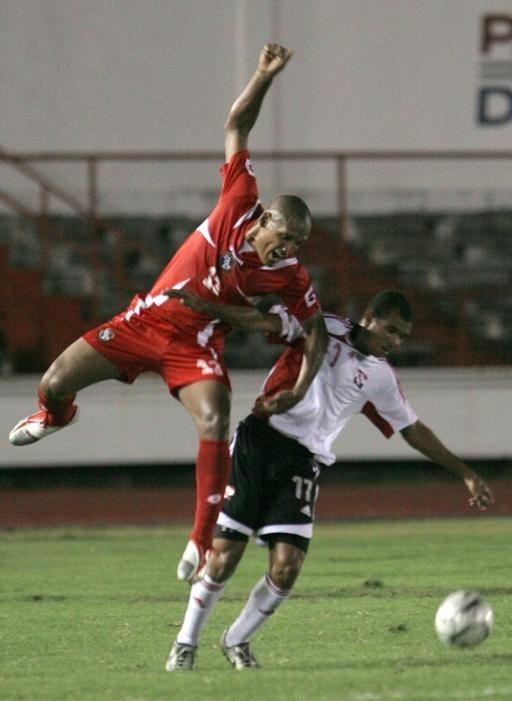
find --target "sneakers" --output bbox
[176,534,212,583]
[8,401,79,445]
[221,628,259,671]
[164,639,197,672]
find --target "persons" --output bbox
[9,42,328,583]
[162,289,495,673]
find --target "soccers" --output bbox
[436,589,492,648]
